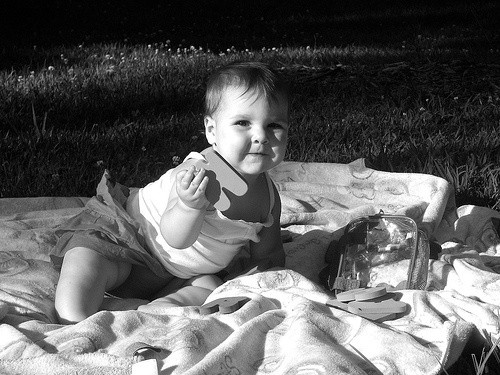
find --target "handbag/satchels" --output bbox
[325,209,442,297]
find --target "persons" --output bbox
[53,58,290,326]
